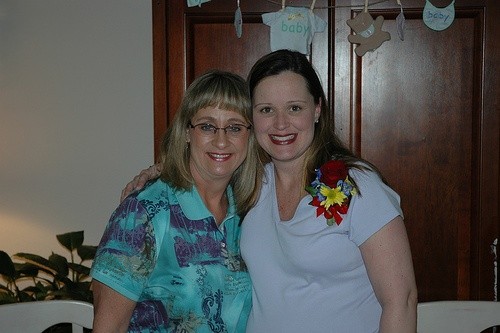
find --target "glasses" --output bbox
[188,118,252,141]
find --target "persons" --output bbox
[89,70,269,333]
[122,50,417,333]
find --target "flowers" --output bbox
[304,159,355,225]
